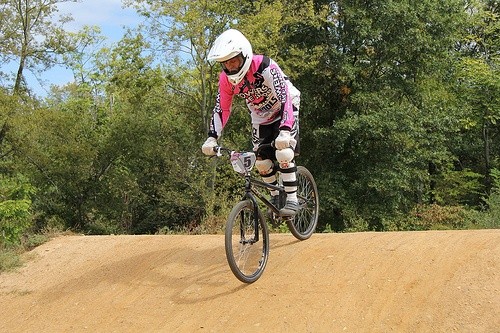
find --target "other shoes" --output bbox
[279,199,300,216]
[267,194,280,213]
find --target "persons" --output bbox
[201,29,302,216]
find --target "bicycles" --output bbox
[208,140,320,283]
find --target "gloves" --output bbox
[202,136,218,155]
[275,129,290,150]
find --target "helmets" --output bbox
[207,28,252,85]
